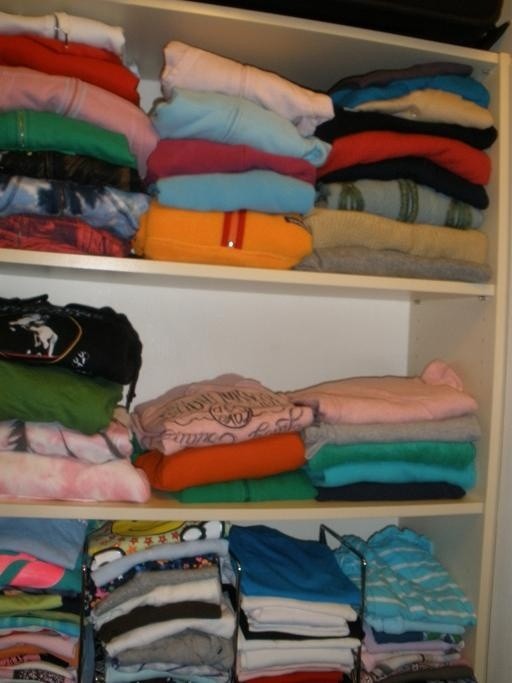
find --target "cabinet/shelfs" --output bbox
[0,0,512,683]
